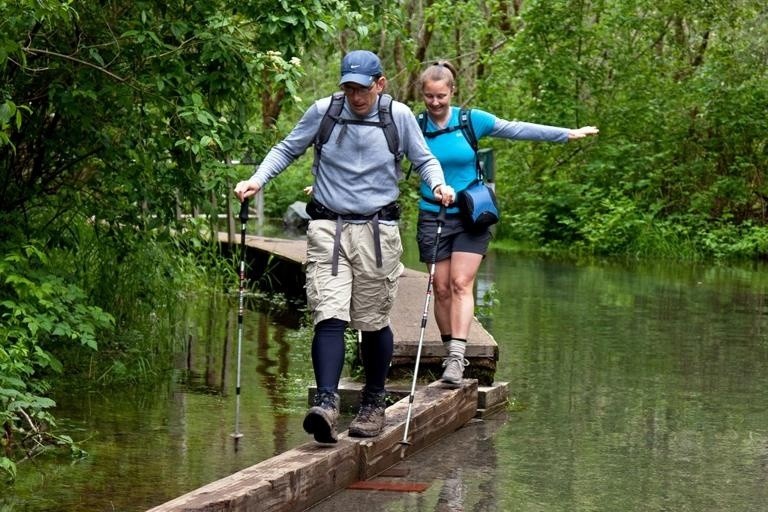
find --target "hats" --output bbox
[338,50,383,87]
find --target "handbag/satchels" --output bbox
[456,181,499,230]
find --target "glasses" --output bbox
[344,81,377,96]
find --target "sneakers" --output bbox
[303,387,340,444]
[442,357,465,384]
[348,390,386,437]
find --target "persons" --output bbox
[304,60,601,385]
[233,49,456,447]
